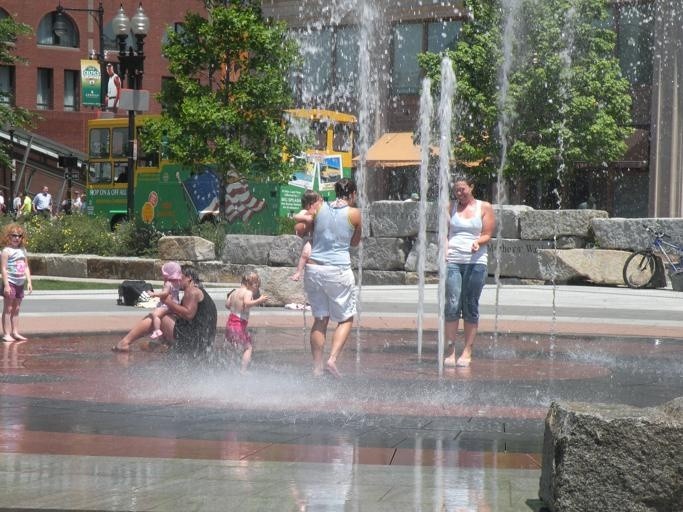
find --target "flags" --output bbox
[183,161,266,226]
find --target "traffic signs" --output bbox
[65,173,81,181]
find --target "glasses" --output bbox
[11,234,23,239]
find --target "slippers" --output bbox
[112,345,129,353]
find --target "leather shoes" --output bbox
[456,356,472,367]
[444,357,456,368]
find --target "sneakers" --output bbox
[151,330,163,340]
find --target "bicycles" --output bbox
[621,223,683,290]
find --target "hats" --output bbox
[162,261,182,281]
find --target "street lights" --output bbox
[8,122,16,218]
[112,0,150,228]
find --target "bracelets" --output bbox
[106,95,109,97]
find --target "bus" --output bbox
[85,107,357,232]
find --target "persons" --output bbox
[290,189,324,283]
[578,195,597,211]
[104,63,122,111]
[61,190,87,215]
[224,272,269,372]
[442,174,495,369]
[109,264,218,355]
[0,222,33,342]
[405,192,419,202]
[293,176,362,379]
[148,260,182,341]
[0,185,53,231]
